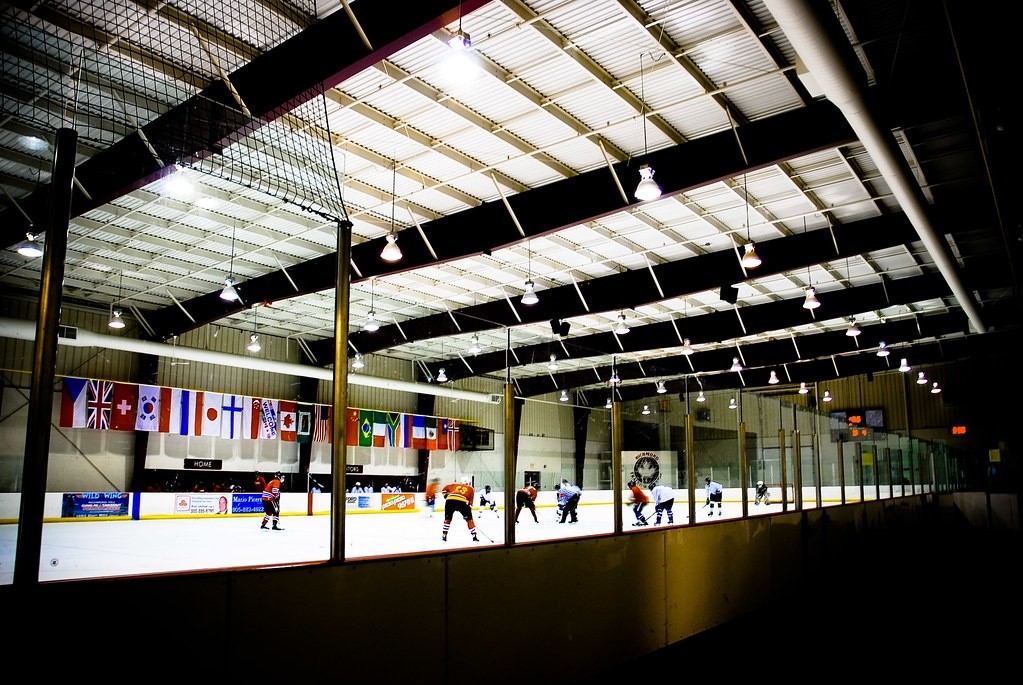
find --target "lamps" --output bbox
[352,324,365,368]
[364,280,379,331]
[448,0,472,50]
[18,133,51,150]
[247,307,262,352]
[521,239,742,414]
[379,160,403,261]
[107,269,126,328]
[469,295,481,353]
[741,161,942,401]
[16,157,43,258]
[633,52,663,200]
[437,342,448,382]
[220,226,239,301]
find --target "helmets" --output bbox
[355,482,361,487]
[628,481,636,489]
[485,485,491,492]
[534,483,540,490]
[757,481,763,488]
[463,479,471,487]
[275,471,284,480]
[705,477,711,482]
[555,485,560,489]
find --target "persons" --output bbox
[425,477,440,504]
[440,479,480,543]
[648,482,676,526]
[625,480,649,526]
[254,471,267,491]
[216,496,228,514]
[479,485,500,518]
[345,482,374,493]
[310,481,323,493]
[514,483,540,523]
[380,483,402,493]
[260,471,285,530]
[555,478,582,524]
[703,477,723,517]
[754,481,770,505]
[191,480,246,493]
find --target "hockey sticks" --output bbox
[474,525,495,543]
[645,511,656,520]
[687,500,710,518]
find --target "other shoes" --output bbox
[260,526,270,531]
[272,526,281,532]
[473,537,479,542]
[442,535,447,542]
[559,520,565,524]
[654,520,661,526]
[535,520,540,523]
[668,520,673,524]
[478,515,482,518]
[569,520,577,524]
[718,512,721,516]
[708,513,713,516]
[497,515,500,518]
[632,522,648,525]
[516,520,520,524]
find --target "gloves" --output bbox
[706,500,710,505]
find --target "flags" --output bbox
[358,411,458,452]
[328,407,358,446]
[277,401,330,443]
[58,377,278,440]
